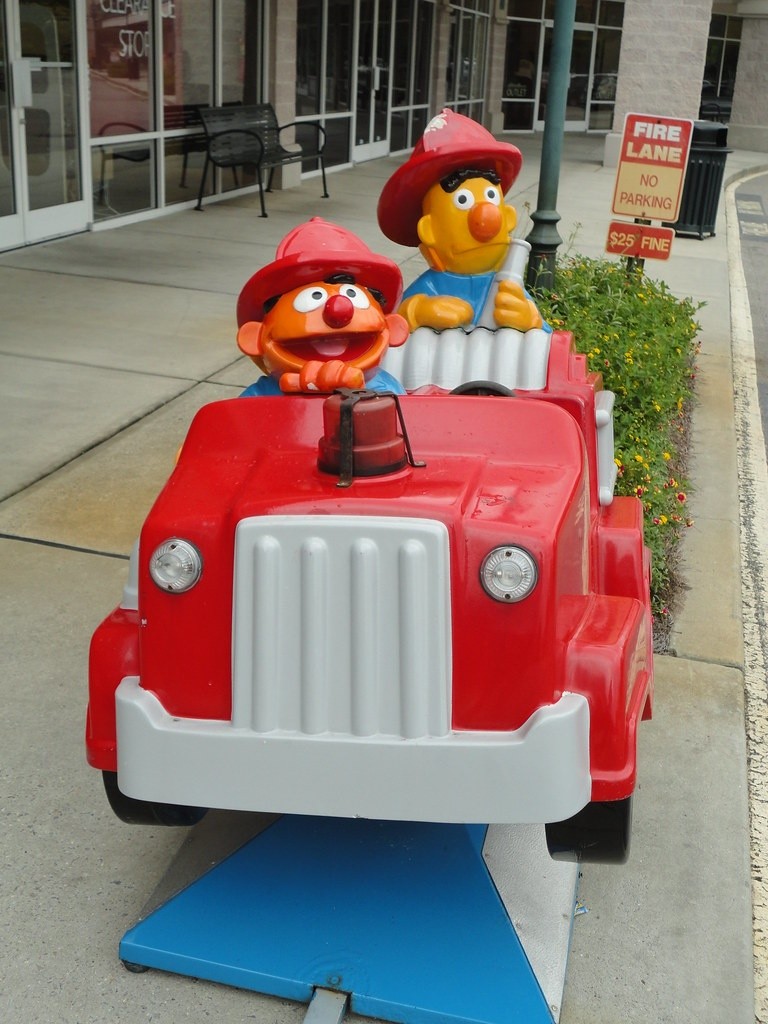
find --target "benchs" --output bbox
[94,101,241,197]
[193,103,330,219]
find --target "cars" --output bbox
[87,328,653,863]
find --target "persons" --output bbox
[229,217,411,396]
[376,109,552,334]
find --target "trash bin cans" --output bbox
[662,119,731,238]
